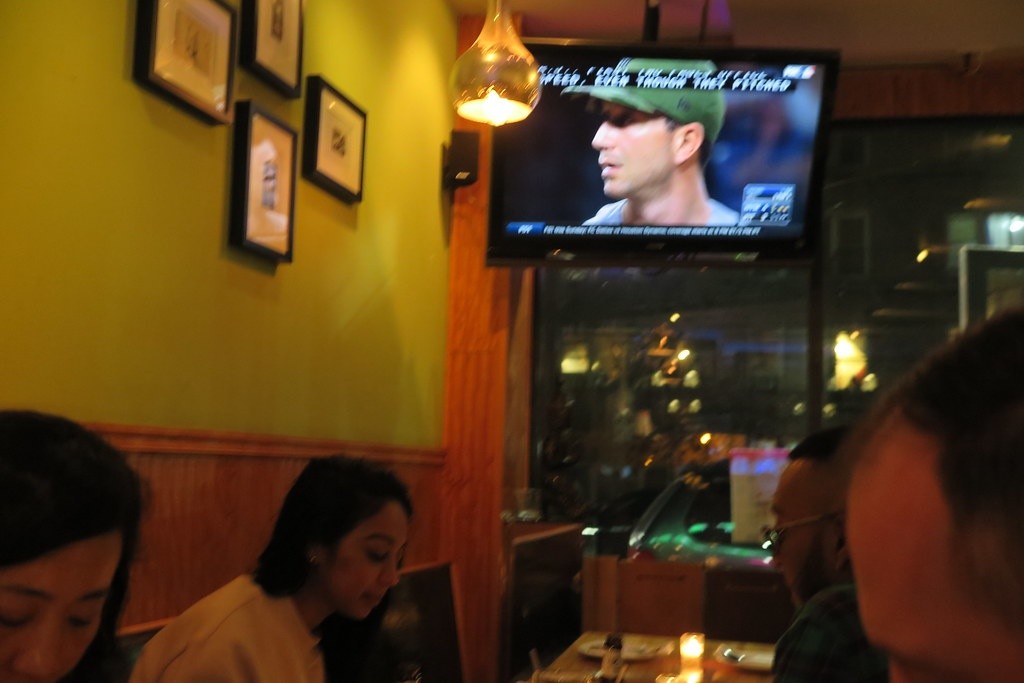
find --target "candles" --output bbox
[680,632,705,665]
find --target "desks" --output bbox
[572,540,796,642]
[529,630,777,683]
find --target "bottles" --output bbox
[588,633,627,683]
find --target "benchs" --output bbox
[498,523,583,683]
[117,560,464,683]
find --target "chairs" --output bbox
[582,556,704,639]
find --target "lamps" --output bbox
[448,0,543,127]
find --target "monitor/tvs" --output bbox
[485,36,841,258]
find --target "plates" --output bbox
[576,638,656,661]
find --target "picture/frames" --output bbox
[301,73,366,206]
[131,0,238,126]
[237,0,304,99]
[227,100,298,265]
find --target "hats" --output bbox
[559,57,726,145]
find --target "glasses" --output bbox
[760,508,834,555]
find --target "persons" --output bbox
[761,306,1024,683]
[559,59,740,227]
[0,410,146,683]
[122,453,414,683]
